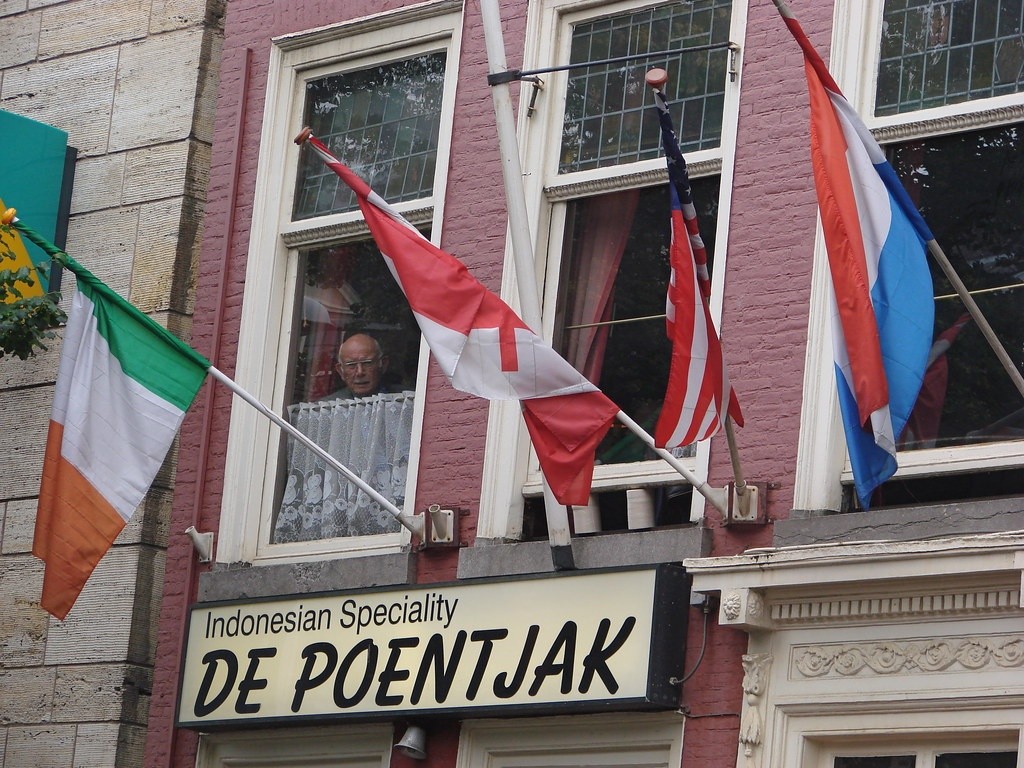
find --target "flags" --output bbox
[650,93,745,448]
[308,137,622,507]
[12,224,209,617]
[785,19,935,506]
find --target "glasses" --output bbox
[343,358,380,369]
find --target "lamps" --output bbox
[394,726,428,761]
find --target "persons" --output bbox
[320,333,410,400]
